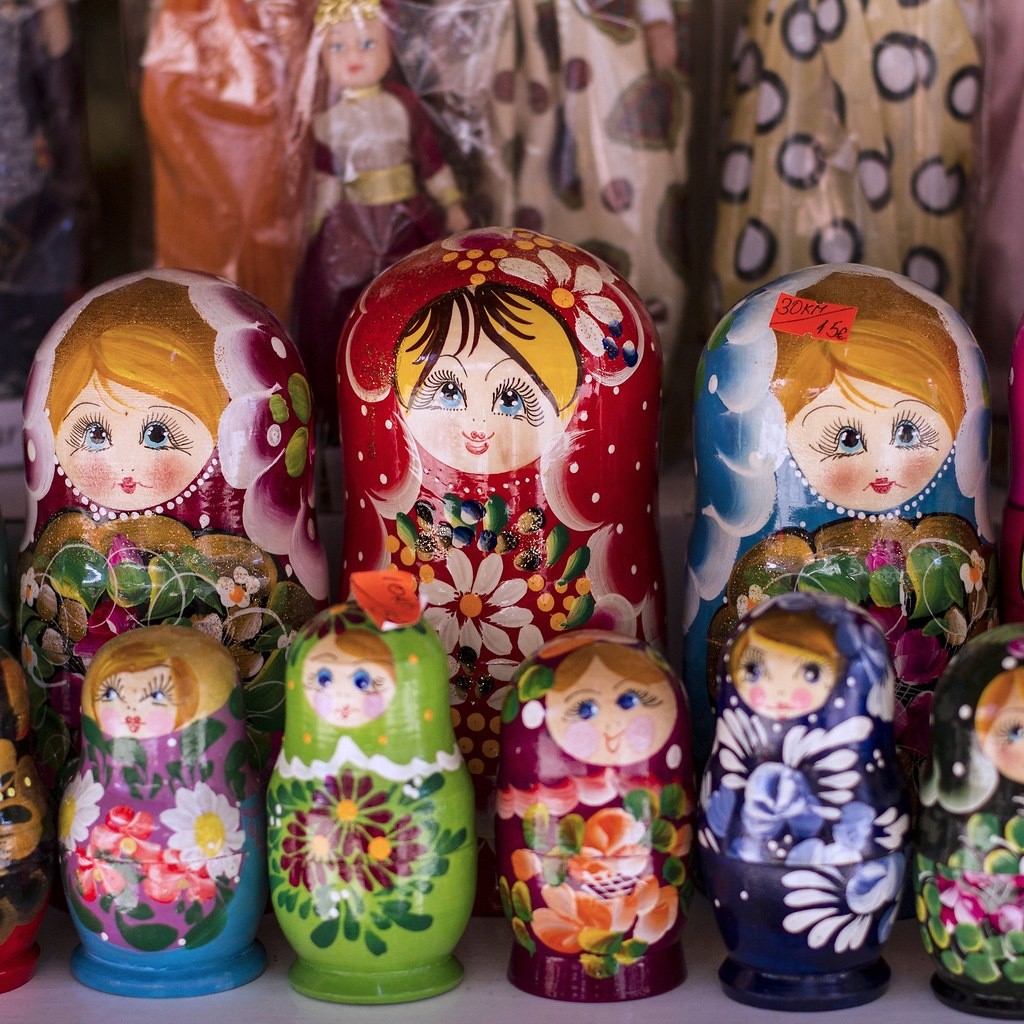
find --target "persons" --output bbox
[286,0,470,449]
